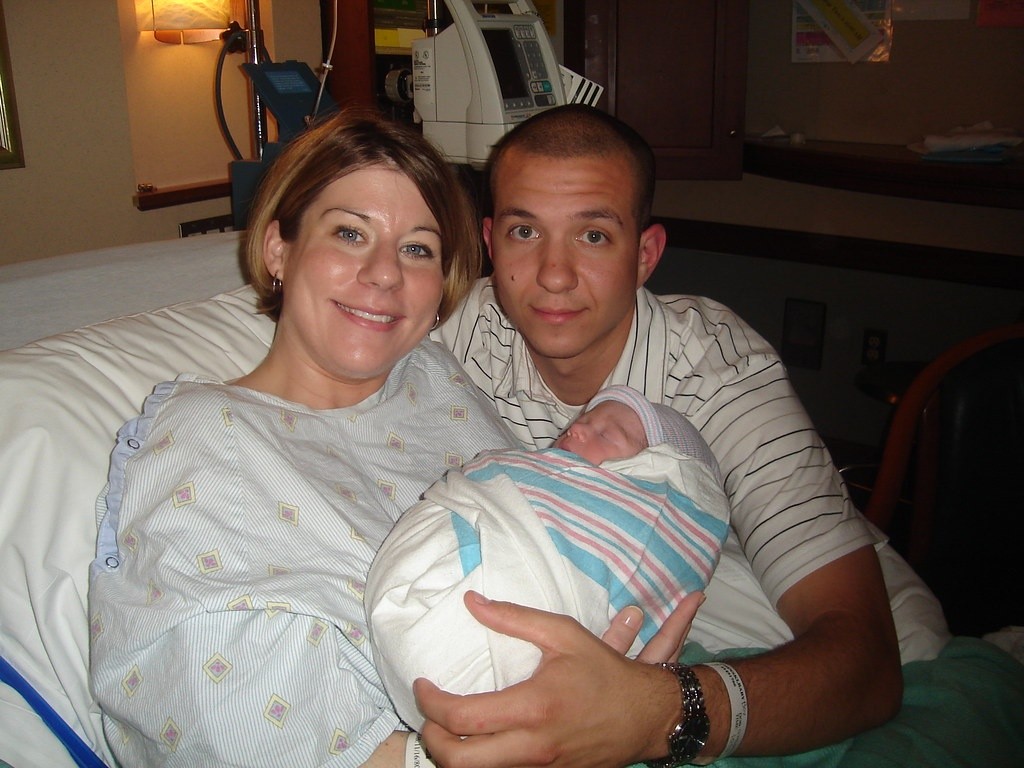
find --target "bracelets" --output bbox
[688,662,748,765]
[405,731,437,768]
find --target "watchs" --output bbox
[642,661,710,768]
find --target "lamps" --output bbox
[150,0,246,45]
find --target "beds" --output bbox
[0,231,1024,768]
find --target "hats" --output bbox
[586,383,722,489]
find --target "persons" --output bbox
[363,382,722,735]
[84,110,705,768]
[412,104,952,768]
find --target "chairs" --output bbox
[840,323,1024,638]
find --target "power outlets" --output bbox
[862,328,887,364]
